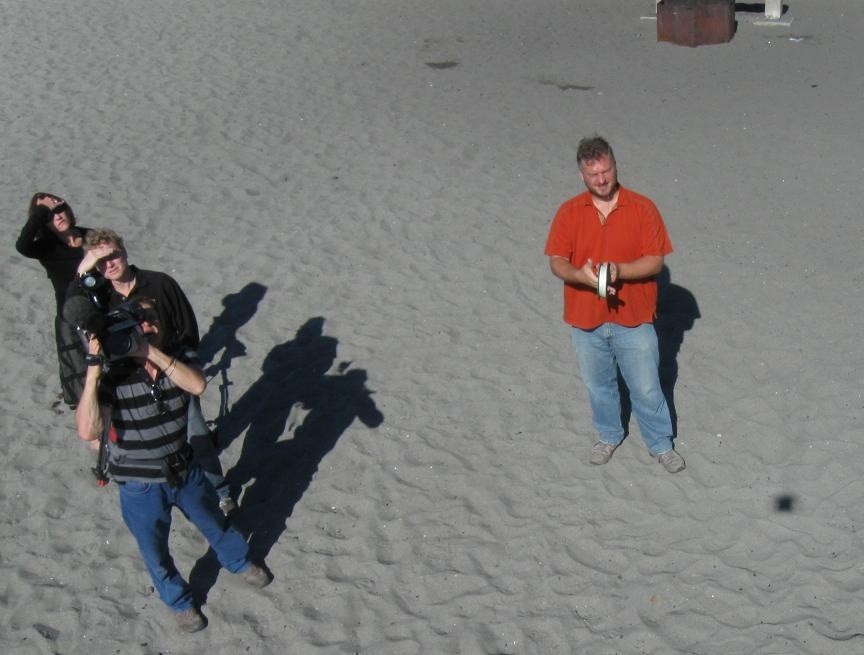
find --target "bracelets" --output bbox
[161,356,176,371]
[166,359,176,378]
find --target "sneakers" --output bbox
[589,440,619,465]
[649,446,686,474]
[236,563,271,588]
[173,604,206,634]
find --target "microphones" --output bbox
[61,294,107,334]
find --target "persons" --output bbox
[546,134,688,477]
[64,226,237,514]
[14,191,98,410]
[72,294,274,632]
